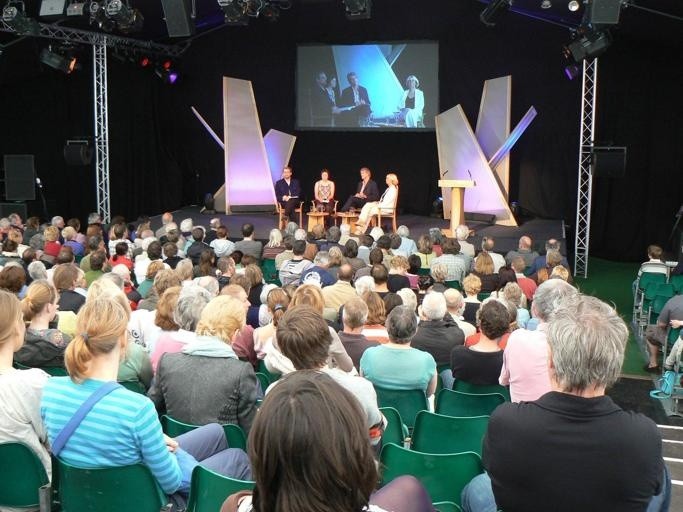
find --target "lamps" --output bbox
[479,0,626,81]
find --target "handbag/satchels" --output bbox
[660,370,683,396]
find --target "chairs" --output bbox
[275,195,304,230]
[305,87,426,130]
[310,198,338,229]
[370,183,400,232]
[349,205,375,228]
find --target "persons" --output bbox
[274,165,303,223]
[0,211,671,509]
[340,72,372,126]
[350,172,399,236]
[313,167,336,227]
[325,75,337,105]
[339,166,380,212]
[311,70,360,126]
[396,75,425,128]
[630,240,683,389]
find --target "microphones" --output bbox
[468,170,472,178]
[443,170,448,176]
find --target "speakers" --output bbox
[161,0,193,37]
[590,0,622,24]
[62,145,91,165]
[4,154,36,201]
[0,203,27,224]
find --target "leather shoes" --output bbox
[643,362,662,375]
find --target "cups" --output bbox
[309,206,312,213]
[318,206,323,213]
[348,208,354,216]
